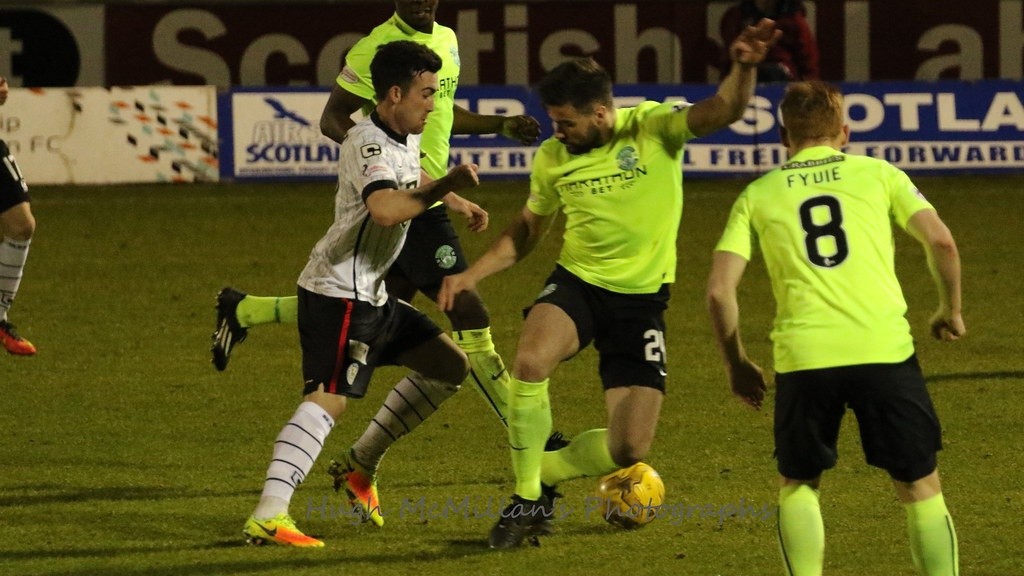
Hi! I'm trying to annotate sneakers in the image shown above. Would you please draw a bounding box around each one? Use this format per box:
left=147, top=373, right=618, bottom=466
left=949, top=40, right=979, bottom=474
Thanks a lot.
left=243, top=512, right=325, bottom=551
left=210, top=287, right=248, bottom=373
left=329, top=451, right=383, bottom=526
left=0, top=321, right=38, bottom=356
left=490, top=492, right=560, bottom=548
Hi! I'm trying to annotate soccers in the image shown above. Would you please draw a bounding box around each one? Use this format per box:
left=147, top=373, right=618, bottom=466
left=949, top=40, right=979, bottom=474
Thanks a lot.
left=599, top=462, right=666, bottom=529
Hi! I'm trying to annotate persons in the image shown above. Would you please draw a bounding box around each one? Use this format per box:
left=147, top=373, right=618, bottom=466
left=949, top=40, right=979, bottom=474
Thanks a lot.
left=704, top=76, right=969, bottom=576
left=0, top=77, right=41, bottom=359
left=209, top=0, right=578, bottom=451
left=233, top=39, right=490, bottom=549
left=719, top=0, right=820, bottom=80
left=436, top=21, right=780, bottom=551
